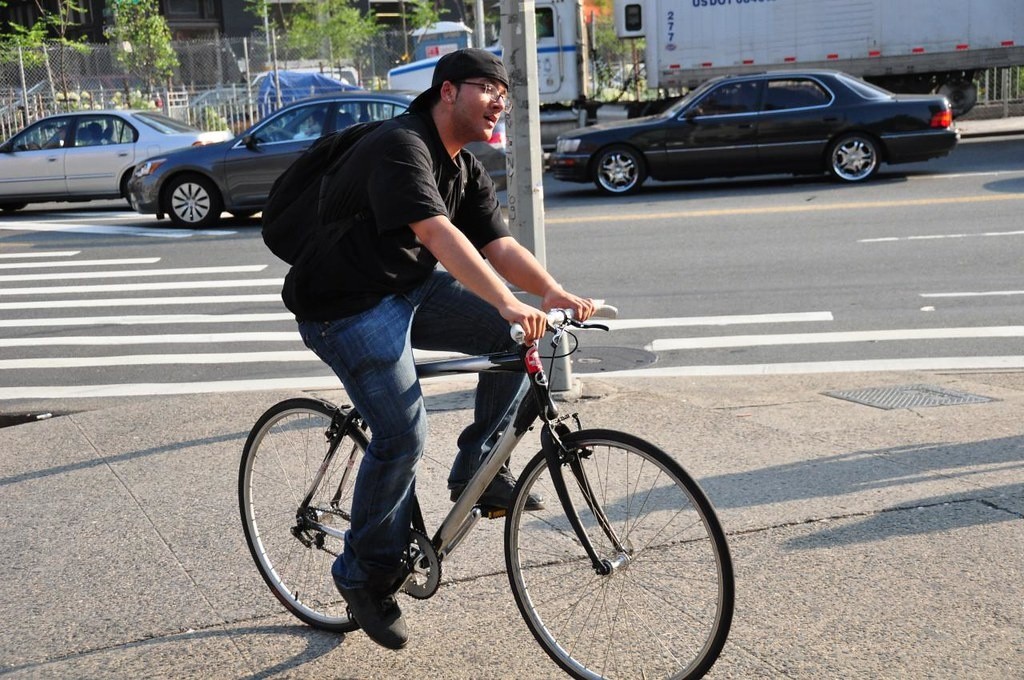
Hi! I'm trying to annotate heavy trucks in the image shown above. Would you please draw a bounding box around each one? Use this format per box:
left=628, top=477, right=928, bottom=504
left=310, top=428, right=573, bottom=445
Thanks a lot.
left=387, top=0, right=1024, bottom=149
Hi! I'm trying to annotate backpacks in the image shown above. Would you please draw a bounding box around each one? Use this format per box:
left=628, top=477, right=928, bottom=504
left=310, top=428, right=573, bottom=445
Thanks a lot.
left=262, top=117, right=438, bottom=265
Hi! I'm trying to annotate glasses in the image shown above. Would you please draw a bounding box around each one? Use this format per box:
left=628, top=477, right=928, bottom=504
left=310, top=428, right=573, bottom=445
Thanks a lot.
left=455, top=80, right=509, bottom=107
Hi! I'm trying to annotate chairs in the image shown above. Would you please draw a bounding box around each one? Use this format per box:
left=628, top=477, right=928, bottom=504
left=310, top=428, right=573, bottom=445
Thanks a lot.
left=77, top=128, right=92, bottom=145
left=337, top=112, right=356, bottom=131
left=739, top=85, right=761, bottom=111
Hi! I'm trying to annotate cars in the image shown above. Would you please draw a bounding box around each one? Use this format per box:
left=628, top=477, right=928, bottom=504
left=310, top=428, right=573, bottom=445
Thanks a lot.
left=547, top=69, right=959, bottom=198
left=126, top=92, right=506, bottom=229
left=0, top=109, right=235, bottom=210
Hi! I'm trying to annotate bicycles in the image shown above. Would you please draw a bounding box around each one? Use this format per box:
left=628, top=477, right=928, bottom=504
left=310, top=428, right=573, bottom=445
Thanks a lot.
left=238, top=297, right=735, bottom=680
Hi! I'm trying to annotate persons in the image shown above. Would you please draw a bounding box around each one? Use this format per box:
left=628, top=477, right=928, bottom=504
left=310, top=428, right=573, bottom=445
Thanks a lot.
left=282, top=49, right=597, bottom=649
left=26, top=123, right=103, bottom=151
left=268, top=111, right=324, bottom=141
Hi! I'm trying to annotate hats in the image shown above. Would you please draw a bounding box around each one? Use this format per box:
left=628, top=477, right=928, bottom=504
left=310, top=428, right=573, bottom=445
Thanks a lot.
left=409, top=48, right=509, bottom=115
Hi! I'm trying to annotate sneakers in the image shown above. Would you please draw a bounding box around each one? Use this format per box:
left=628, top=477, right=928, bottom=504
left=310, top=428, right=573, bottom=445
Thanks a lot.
left=450, top=468, right=544, bottom=510
left=340, top=581, right=409, bottom=648
